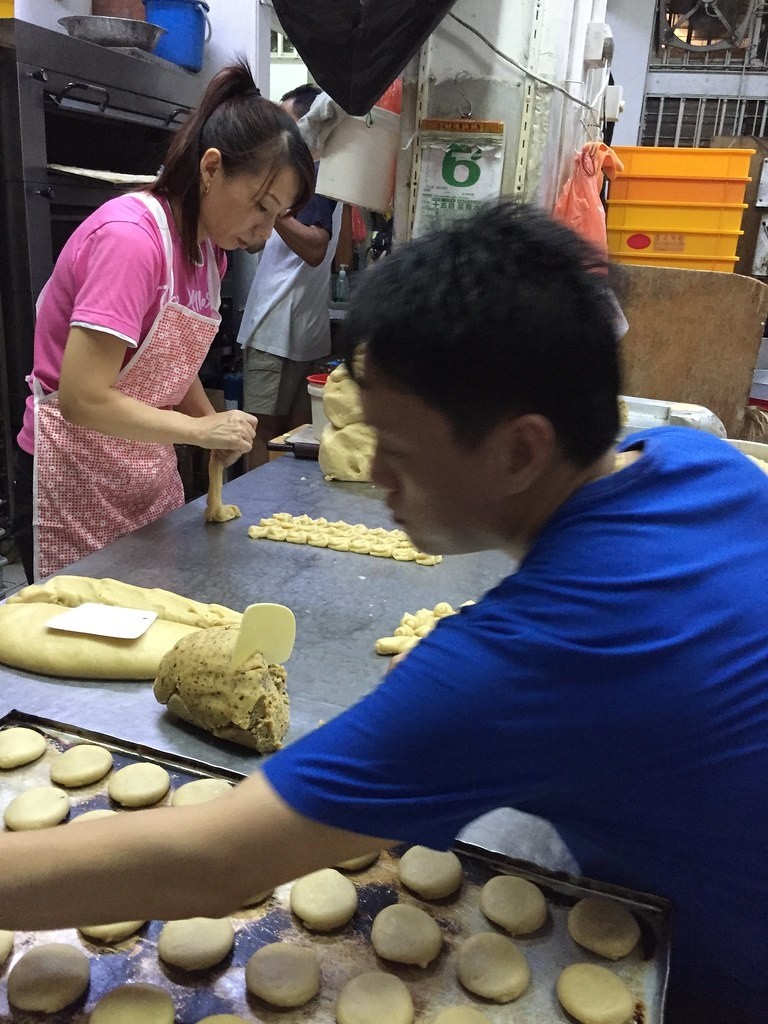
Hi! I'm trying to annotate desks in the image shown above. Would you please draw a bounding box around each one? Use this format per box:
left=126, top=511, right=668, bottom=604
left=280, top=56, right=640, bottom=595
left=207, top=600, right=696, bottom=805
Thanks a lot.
left=0, top=454, right=576, bottom=889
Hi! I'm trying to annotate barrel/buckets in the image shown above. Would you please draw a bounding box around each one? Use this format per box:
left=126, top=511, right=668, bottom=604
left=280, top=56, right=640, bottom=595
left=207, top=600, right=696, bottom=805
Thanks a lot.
left=141, top=1, right=211, bottom=72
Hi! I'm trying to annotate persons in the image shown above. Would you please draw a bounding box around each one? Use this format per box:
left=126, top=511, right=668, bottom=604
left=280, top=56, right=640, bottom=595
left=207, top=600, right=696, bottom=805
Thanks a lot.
left=11, top=57, right=316, bottom=587
left=1, top=197, right=766, bottom=1023
left=236, top=83, right=345, bottom=453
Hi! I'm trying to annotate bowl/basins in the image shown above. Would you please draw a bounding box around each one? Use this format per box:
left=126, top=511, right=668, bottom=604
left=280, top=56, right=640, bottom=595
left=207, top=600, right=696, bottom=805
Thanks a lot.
left=58, top=16, right=169, bottom=53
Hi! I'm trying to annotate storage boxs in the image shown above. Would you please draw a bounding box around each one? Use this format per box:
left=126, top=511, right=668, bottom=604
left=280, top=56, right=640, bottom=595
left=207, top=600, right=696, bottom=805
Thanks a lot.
left=606, top=250, right=741, bottom=276
left=606, top=172, right=753, bottom=205
left=604, top=226, right=745, bottom=257
left=609, top=144, right=756, bottom=179
left=602, top=198, right=748, bottom=232
